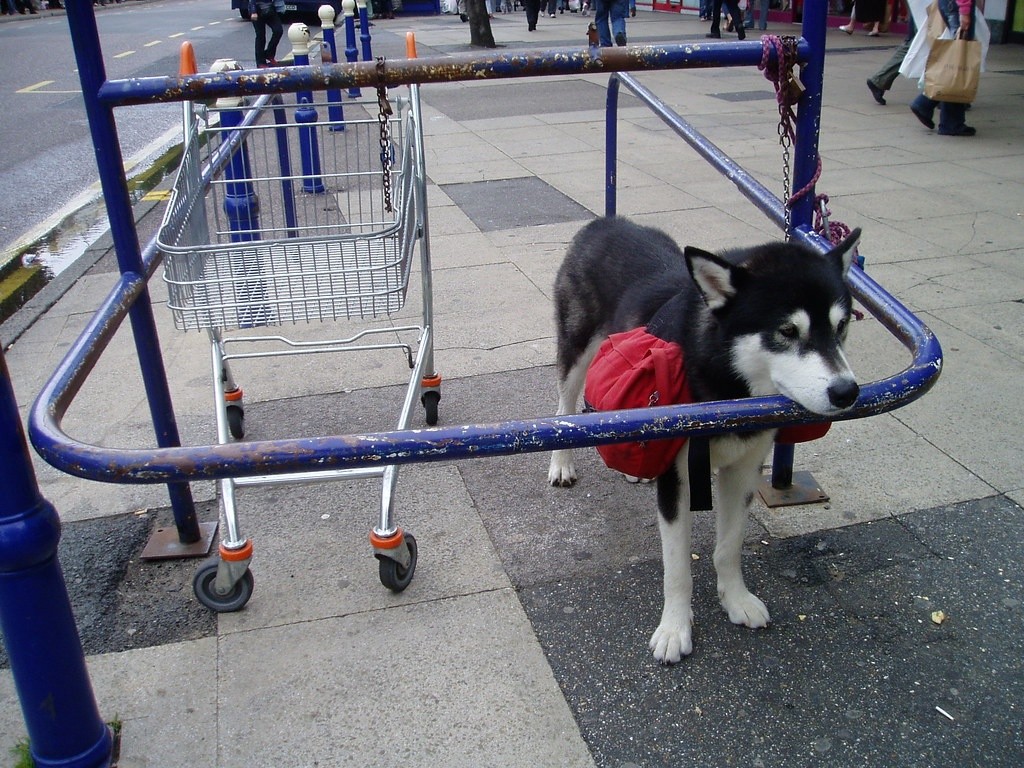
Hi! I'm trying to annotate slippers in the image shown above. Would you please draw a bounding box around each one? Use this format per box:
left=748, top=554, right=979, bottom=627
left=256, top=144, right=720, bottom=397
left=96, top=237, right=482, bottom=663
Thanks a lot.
left=839, top=25, right=852, bottom=35
left=868, top=31, right=879, bottom=36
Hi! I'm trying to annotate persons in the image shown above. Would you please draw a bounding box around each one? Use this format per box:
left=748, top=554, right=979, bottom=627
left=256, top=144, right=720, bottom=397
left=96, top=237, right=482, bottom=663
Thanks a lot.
left=458, top=0, right=636, bottom=47
left=248, top=0, right=286, bottom=68
left=0, top=0, right=124, bottom=14
left=699, top=0, right=769, bottom=40
left=838, top=0, right=979, bottom=136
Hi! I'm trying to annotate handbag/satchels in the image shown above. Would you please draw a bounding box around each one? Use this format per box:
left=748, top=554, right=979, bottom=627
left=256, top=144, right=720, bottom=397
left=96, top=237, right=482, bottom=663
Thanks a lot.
left=585, top=327, right=695, bottom=478
left=772, top=422, right=831, bottom=444
left=923, top=27, right=982, bottom=103
left=738, top=0, right=750, bottom=12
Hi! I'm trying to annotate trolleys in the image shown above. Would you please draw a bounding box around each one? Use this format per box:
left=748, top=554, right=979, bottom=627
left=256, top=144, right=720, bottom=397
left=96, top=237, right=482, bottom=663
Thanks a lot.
left=153, top=30, right=443, bottom=613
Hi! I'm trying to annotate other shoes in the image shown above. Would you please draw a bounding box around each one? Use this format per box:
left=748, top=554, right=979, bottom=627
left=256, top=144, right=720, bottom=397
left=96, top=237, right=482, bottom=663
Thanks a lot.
left=727, top=18, right=735, bottom=32
left=550, top=13, right=555, bottom=18
left=744, top=27, right=753, bottom=30
left=909, top=105, right=935, bottom=130
left=867, top=78, right=886, bottom=106
left=632, top=10, right=636, bottom=17
left=269, top=57, right=280, bottom=67
left=706, top=33, right=721, bottom=38
left=616, top=34, right=626, bottom=46
left=257, top=63, right=271, bottom=68
left=738, top=26, right=745, bottom=40
left=701, top=16, right=707, bottom=21
left=938, top=127, right=976, bottom=136
left=540, top=10, right=545, bottom=17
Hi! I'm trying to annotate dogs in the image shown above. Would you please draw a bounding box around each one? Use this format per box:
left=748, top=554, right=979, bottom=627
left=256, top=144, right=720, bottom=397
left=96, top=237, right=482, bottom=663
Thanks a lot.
left=547, top=216, right=862, bottom=666
